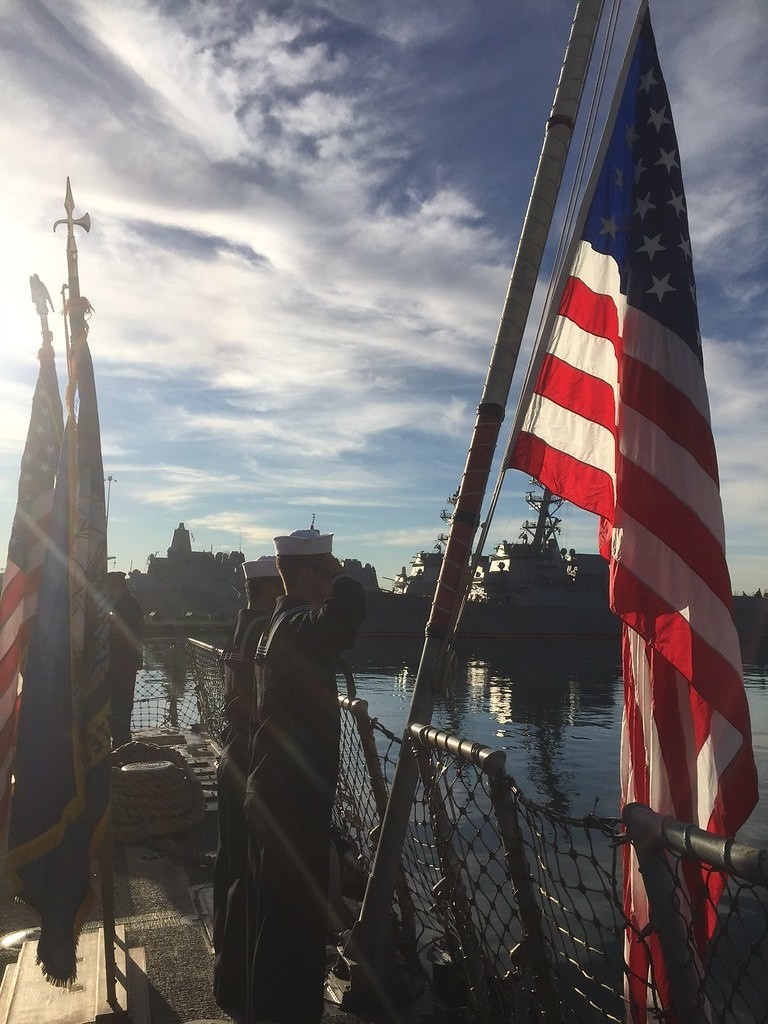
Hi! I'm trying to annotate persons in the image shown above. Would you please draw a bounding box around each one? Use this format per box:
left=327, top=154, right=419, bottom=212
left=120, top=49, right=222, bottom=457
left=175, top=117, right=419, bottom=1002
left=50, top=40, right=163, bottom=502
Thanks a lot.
left=213, top=530, right=366, bottom=1024
left=106, top=572, right=145, bottom=750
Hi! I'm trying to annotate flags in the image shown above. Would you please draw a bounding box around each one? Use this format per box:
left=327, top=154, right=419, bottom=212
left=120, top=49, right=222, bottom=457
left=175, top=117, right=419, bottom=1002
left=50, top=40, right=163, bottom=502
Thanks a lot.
left=506, top=0, right=760, bottom=1024
left=0, top=296, right=112, bottom=990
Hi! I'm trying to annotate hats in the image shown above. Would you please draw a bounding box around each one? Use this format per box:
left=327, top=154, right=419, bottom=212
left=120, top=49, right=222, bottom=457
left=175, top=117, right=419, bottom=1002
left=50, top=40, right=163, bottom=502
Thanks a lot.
left=274, top=530, right=334, bottom=556
left=241, top=556, right=281, bottom=580
left=107, top=572, right=126, bottom=580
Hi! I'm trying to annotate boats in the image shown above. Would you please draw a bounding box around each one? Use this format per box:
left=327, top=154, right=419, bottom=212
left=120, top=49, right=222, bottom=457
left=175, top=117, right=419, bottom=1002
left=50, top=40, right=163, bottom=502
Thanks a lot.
left=125, top=476, right=623, bottom=667
